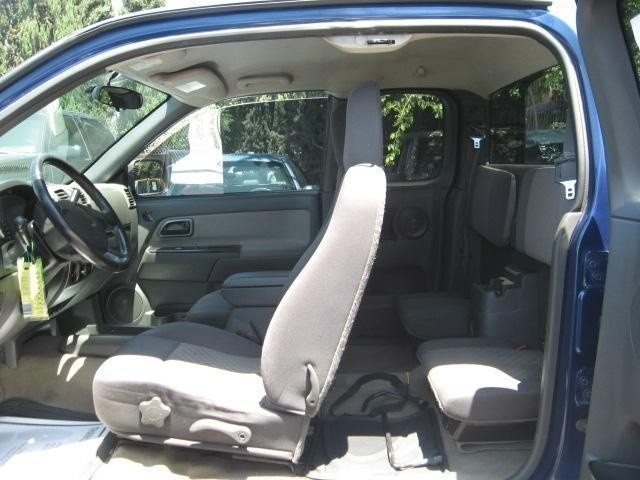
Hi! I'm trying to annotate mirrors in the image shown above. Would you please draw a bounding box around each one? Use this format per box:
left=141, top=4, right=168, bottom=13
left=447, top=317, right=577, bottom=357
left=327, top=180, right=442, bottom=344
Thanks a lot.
left=97, top=85, right=144, bottom=109
